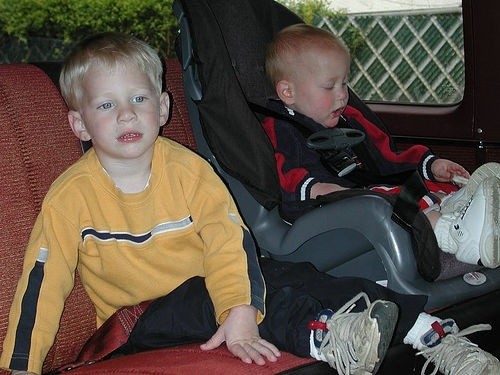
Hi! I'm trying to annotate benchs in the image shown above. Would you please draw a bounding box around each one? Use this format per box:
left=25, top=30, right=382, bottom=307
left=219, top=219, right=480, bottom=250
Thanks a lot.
left=0, top=57, right=500, bottom=375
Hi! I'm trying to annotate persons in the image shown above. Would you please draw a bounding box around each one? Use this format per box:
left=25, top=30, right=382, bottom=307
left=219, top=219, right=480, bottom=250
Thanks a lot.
left=0, top=30, right=500, bottom=375
left=261, top=24, right=499, bottom=268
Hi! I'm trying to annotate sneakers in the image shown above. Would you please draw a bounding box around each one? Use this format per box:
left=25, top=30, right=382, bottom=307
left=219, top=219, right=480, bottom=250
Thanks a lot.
left=308, top=292, right=399, bottom=375
left=440, top=162, right=500, bottom=222
left=449, top=176, right=500, bottom=269
left=416, top=318, right=500, bottom=375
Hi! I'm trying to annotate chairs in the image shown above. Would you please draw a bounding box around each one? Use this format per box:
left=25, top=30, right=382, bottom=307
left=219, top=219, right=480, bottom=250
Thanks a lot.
left=172, top=1, right=500, bottom=346
